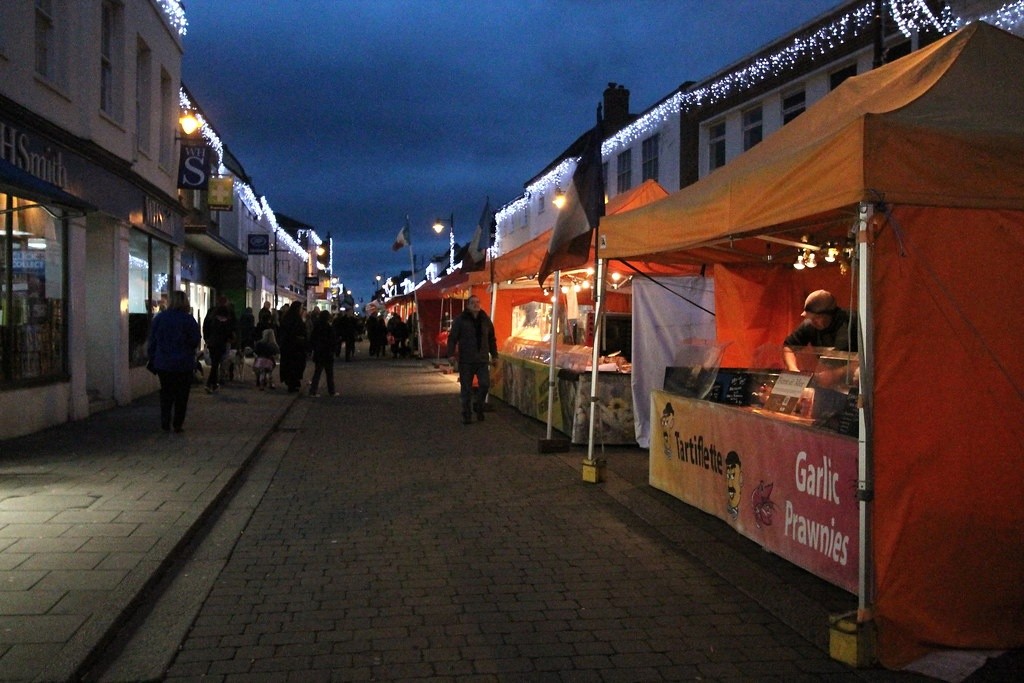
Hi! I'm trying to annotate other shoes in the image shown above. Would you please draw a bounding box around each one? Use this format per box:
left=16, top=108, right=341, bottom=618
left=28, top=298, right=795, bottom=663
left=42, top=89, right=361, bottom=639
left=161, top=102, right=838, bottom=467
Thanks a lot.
left=461, top=411, right=473, bottom=424
left=472, top=401, right=485, bottom=421
left=162, top=422, right=185, bottom=434
left=309, top=393, right=320, bottom=398
left=204, top=382, right=221, bottom=394
left=330, top=392, right=340, bottom=397
left=259, top=383, right=276, bottom=391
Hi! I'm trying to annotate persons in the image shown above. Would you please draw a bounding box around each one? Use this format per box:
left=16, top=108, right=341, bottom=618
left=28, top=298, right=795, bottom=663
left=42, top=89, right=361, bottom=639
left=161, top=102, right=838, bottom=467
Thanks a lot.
left=147, top=291, right=199, bottom=432
left=280, top=301, right=305, bottom=393
left=254, top=329, right=280, bottom=390
left=204, top=305, right=230, bottom=393
left=447, top=295, right=498, bottom=423
left=214, top=297, right=416, bottom=361
left=309, top=311, right=339, bottom=398
left=783, top=290, right=860, bottom=384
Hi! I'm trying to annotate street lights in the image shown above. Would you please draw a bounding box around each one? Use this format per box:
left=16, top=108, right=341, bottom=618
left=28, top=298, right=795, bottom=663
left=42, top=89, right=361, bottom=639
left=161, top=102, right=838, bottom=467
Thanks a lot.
left=340, top=272, right=382, bottom=316
left=431, top=212, right=456, bottom=274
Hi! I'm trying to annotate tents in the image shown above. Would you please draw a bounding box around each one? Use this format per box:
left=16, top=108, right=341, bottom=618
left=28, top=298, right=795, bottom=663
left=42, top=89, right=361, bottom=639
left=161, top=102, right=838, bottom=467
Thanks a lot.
left=491, top=177, right=670, bottom=444
left=583, top=20, right=1022, bottom=664
left=385, top=269, right=490, bottom=360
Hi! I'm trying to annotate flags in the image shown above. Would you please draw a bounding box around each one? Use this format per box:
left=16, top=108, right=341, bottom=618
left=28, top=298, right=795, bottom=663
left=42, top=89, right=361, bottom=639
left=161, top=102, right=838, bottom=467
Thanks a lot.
left=462, top=201, right=489, bottom=270
left=392, top=221, right=410, bottom=252
left=537, top=124, right=603, bottom=287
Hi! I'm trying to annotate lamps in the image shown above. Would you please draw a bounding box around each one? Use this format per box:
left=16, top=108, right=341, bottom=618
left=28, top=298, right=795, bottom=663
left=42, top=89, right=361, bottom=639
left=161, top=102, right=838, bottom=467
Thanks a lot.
left=805, top=249, right=818, bottom=268
left=825, top=240, right=838, bottom=263
left=180, top=109, right=199, bottom=134
left=843, top=238, right=855, bottom=259
left=792, top=251, right=806, bottom=271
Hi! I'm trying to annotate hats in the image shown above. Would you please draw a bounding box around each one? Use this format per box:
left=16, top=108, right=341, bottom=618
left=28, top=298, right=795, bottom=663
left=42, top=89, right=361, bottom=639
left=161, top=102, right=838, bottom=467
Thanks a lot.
left=800, top=289, right=838, bottom=319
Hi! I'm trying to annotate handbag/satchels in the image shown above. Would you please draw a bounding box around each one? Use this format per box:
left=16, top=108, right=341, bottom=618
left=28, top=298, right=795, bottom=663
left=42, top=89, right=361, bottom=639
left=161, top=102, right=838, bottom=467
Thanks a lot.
left=146, top=340, right=157, bottom=375
